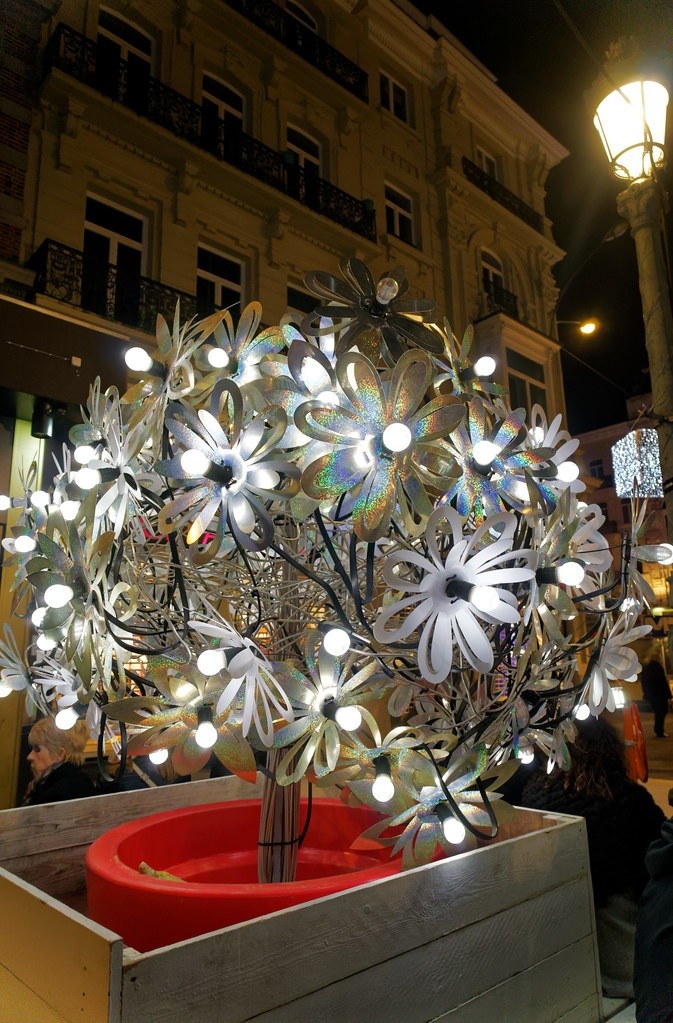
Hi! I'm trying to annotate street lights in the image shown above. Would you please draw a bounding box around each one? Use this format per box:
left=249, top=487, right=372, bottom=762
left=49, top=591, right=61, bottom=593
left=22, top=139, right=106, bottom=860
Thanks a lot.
left=586, top=30, right=673, bottom=501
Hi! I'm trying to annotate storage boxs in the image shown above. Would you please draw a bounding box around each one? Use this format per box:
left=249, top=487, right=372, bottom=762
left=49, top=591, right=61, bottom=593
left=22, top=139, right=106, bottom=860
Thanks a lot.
left=1, top=769, right=603, bottom=1023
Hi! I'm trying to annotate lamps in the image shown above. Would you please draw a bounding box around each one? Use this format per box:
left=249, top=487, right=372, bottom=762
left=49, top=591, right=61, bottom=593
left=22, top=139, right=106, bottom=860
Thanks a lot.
left=0, top=256, right=673, bottom=873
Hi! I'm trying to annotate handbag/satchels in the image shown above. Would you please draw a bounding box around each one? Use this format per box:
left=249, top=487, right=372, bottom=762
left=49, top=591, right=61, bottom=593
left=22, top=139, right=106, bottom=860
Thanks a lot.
left=637, top=699, right=653, bottom=712
left=623, top=703, right=648, bottom=783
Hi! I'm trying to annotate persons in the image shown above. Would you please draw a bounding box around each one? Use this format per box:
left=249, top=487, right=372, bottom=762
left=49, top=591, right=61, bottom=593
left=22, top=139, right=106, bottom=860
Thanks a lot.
left=498, top=713, right=673, bottom=1023
left=24, top=714, right=96, bottom=807
left=639, top=642, right=673, bottom=737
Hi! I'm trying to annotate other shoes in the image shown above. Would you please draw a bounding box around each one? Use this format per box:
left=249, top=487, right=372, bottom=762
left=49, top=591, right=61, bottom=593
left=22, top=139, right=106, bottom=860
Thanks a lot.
left=657, top=733, right=669, bottom=738
